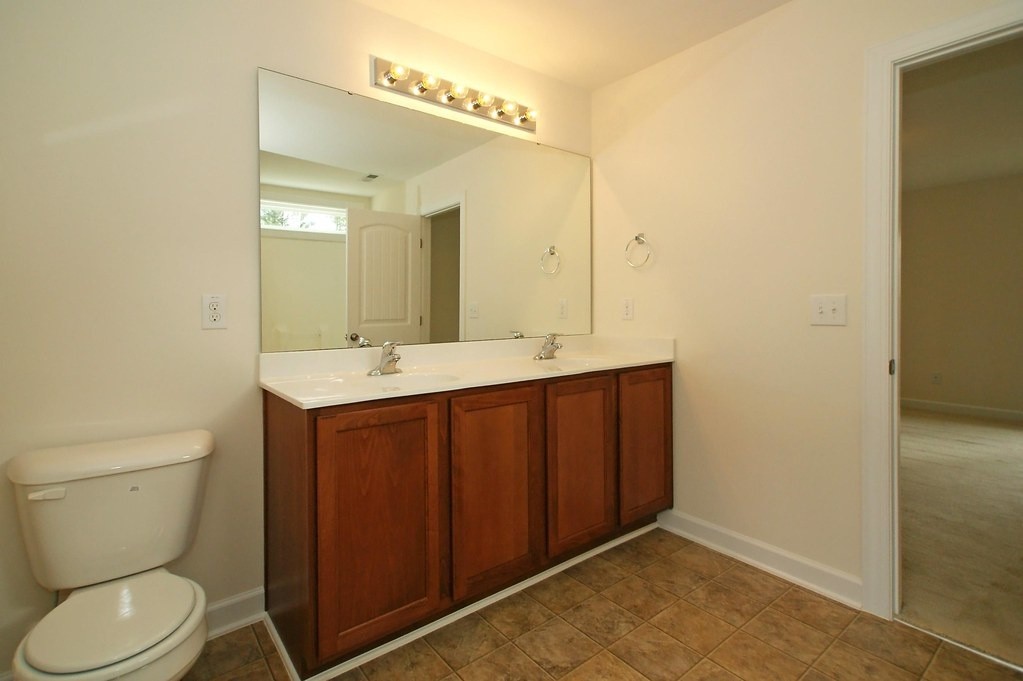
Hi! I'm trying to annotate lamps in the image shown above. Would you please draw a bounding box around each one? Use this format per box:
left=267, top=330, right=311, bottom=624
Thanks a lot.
left=373, top=58, right=537, bottom=131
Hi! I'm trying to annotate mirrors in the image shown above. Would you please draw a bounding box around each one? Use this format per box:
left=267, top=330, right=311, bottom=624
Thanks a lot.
left=255, top=65, right=593, bottom=353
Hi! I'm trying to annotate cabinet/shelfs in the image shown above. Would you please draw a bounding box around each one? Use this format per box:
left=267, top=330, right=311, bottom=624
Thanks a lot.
left=545, top=360, right=673, bottom=572
left=262, top=379, right=546, bottom=681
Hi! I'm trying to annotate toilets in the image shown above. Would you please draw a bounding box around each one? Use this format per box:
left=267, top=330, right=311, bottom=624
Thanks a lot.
left=6, top=430, right=214, bottom=681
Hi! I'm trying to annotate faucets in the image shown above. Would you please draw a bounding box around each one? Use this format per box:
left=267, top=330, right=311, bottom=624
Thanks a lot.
left=358, top=337, right=371, bottom=347
left=533, top=333, right=563, bottom=360
left=367, top=342, right=401, bottom=376
left=510, top=331, right=524, bottom=338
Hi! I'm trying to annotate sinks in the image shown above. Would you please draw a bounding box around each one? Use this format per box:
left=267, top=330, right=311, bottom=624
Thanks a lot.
left=501, top=356, right=618, bottom=376
left=329, top=374, right=459, bottom=392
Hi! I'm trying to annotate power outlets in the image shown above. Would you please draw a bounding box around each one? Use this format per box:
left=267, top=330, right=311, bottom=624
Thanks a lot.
left=201, top=293, right=228, bottom=330
left=931, top=371, right=943, bottom=385
left=557, top=299, right=568, bottom=319
left=620, top=296, right=633, bottom=319
